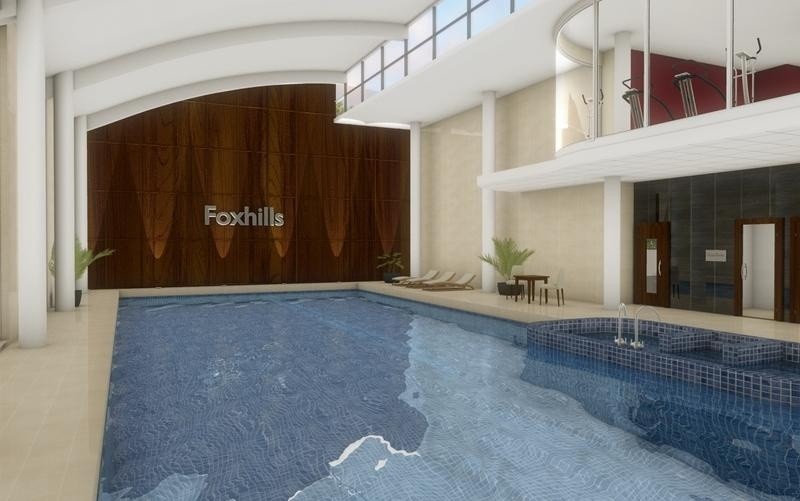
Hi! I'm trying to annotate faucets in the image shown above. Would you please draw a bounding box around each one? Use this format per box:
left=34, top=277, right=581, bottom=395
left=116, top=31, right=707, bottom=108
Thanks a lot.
left=614, top=303, right=628, bottom=346
left=630, top=305, right=662, bottom=349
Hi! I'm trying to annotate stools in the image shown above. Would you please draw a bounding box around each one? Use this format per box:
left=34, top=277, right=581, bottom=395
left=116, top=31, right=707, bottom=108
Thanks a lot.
left=497, top=282, right=525, bottom=299
left=537, top=283, right=565, bottom=307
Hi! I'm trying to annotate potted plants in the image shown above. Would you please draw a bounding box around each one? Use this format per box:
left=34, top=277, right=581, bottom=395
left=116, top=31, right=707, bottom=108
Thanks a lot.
left=377, top=252, right=403, bottom=283
left=49, top=232, right=113, bottom=306
left=479, top=237, right=536, bottom=295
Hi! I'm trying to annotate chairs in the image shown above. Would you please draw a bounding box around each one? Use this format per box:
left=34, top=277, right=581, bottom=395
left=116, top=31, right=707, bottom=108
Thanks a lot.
left=392, top=270, right=476, bottom=290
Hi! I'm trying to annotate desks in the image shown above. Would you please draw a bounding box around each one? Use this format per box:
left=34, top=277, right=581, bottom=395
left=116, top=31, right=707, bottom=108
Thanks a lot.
left=513, top=275, right=550, bottom=304
